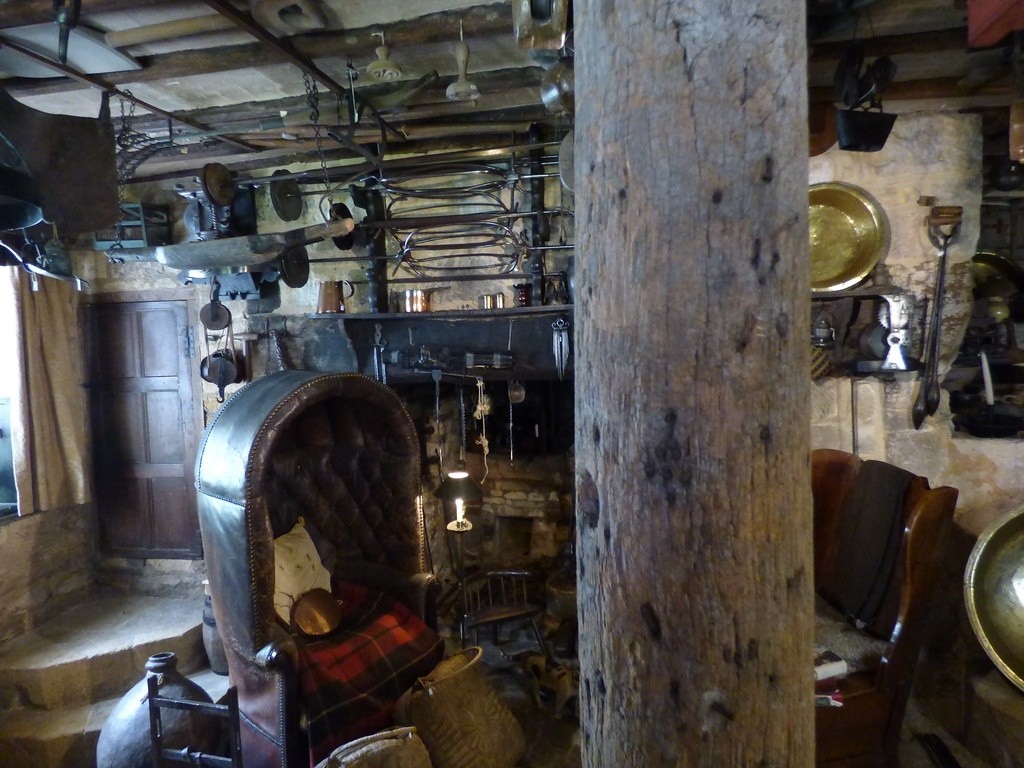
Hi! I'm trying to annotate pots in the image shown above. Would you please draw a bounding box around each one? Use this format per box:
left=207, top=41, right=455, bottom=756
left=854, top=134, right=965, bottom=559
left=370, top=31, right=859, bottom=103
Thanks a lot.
left=834, top=105, right=897, bottom=152
left=397, top=286, right=455, bottom=312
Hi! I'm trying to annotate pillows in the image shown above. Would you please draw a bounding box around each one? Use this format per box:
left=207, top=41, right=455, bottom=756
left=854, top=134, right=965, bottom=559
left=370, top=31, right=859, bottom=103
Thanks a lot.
left=273, top=516, right=332, bottom=625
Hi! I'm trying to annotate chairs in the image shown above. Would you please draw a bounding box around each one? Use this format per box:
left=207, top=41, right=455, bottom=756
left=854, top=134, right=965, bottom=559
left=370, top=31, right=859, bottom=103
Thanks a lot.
left=147, top=675, right=243, bottom=768
left=457, top=569, right=548, bottom=655
left=193, top=370, right=447, bottom=768
left=809, top=447, right=958, bottom=768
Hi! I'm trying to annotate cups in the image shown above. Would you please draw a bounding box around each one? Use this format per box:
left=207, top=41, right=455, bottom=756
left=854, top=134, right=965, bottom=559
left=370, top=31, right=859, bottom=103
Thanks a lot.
left=482, top=293, right=503, bottom=309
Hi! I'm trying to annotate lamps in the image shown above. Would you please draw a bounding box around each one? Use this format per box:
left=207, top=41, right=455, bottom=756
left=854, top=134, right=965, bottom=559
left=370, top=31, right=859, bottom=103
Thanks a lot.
left=446, top=445, right=469, bottom=480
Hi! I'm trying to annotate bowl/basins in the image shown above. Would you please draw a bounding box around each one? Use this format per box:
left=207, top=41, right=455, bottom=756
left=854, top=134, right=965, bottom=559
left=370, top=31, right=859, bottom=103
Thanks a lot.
left=965, top=503, right=1023, bottom=692
left=974, top=251, right=1022, bottom=315
left=807, top=182, right=886, bottom=291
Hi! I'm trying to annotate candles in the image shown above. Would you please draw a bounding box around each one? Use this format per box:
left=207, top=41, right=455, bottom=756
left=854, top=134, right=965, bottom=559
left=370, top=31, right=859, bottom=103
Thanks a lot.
left=455, top=498, right=464, bottom=522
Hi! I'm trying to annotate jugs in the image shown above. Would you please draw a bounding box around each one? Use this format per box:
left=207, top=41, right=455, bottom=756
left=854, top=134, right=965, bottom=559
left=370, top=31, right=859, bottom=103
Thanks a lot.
left=317, top=279, right=355, bottom=313
